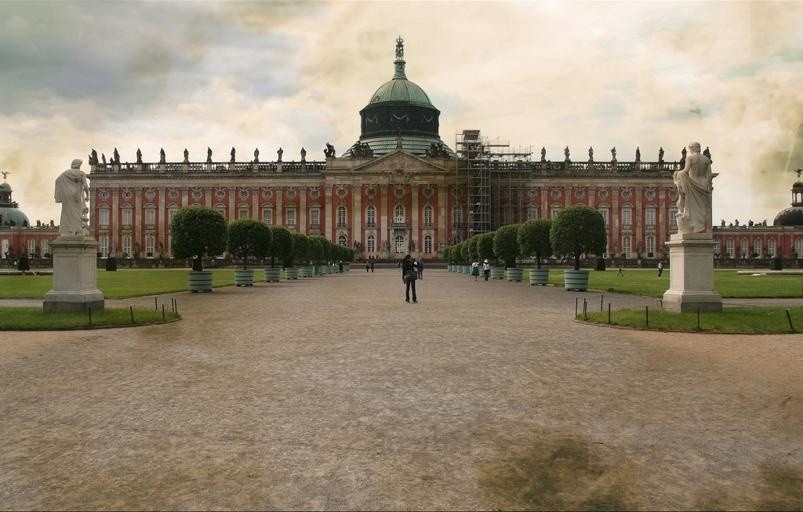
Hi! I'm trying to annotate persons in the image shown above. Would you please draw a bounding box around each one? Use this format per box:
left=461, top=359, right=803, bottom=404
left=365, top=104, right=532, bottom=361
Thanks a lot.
left=672, top=142, right=713, bottom=237
left=365, top=260, right=369, bottom=272
left=482, top=259, right=489, bottom=281
left=470, top=259, right=479, bottom=282
left=370, top=260, right=374, bottom=272
left=401, top=254, right=417, bottom=304
left=339, top=260, right=342, bottom=272
left=417, top=257, right=422, bottom=280
left=657, top=259, right=663, bottom=277
left=52, top=158, right=88, bottom=238
left=615, top=262, right=624, bottom=278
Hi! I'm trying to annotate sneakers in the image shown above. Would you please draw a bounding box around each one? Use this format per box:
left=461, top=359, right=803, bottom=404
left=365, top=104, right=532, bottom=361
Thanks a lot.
left=405, top=299, right=418, bottom=303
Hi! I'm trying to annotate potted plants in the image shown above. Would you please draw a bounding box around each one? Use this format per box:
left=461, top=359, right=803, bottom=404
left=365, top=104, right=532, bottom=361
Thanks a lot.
left=443, top=204, right=607, bottom=292
left=168, top=204, right=354, bottom=293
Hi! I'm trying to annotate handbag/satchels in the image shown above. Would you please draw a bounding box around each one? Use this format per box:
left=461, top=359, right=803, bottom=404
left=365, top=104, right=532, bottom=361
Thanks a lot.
left=403, top=269, right=418, bottom=283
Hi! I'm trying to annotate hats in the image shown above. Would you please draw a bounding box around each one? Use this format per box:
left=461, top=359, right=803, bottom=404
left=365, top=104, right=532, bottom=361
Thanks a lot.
left=483, top=259, right=489, bottom=264
